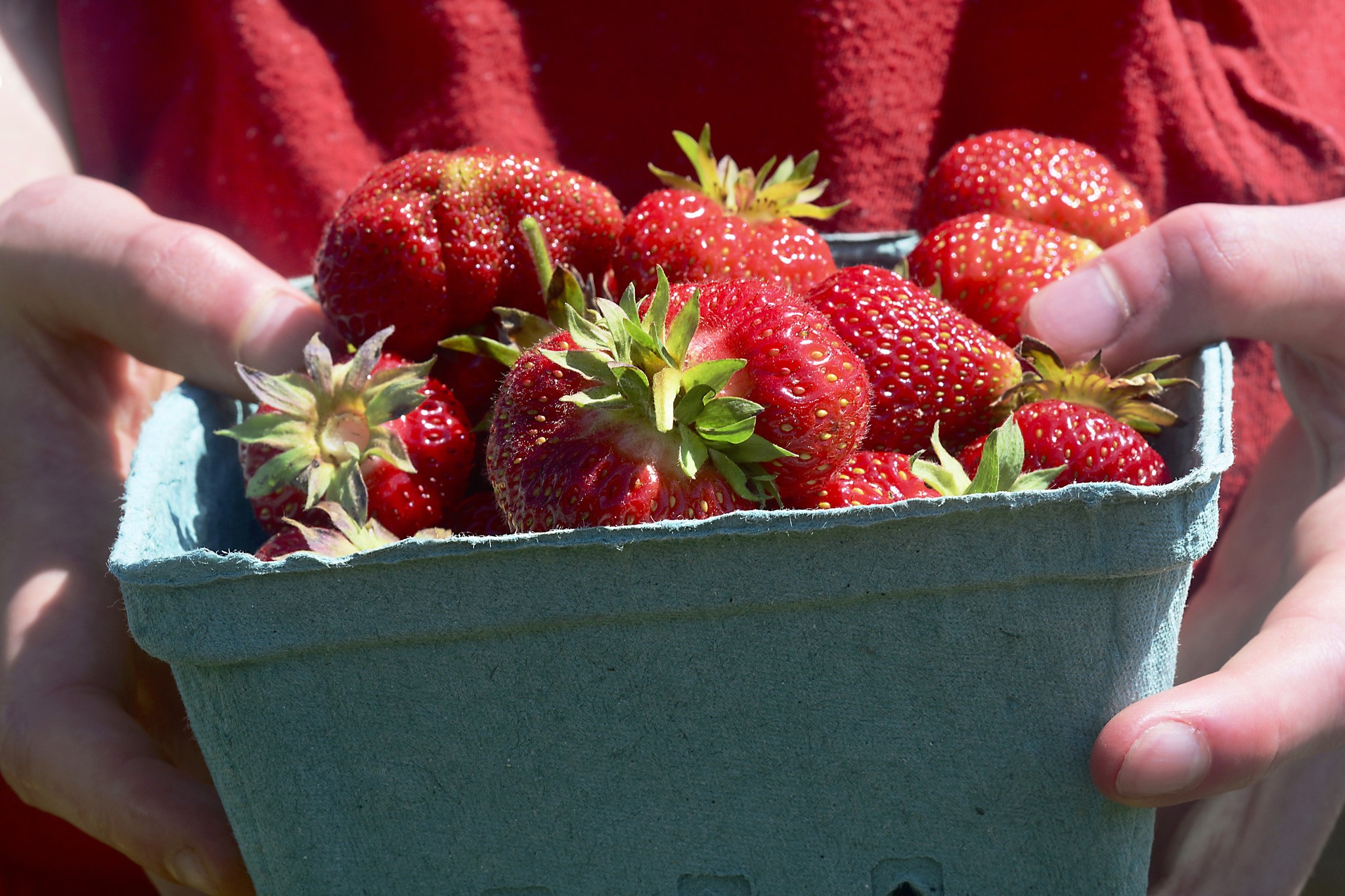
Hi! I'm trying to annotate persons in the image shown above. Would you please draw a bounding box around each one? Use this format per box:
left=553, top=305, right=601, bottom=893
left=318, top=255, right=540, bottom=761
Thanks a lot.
left=0, top=0, right=1345, bottom=896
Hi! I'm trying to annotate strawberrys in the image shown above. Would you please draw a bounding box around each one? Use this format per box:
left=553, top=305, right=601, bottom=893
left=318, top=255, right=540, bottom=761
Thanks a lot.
left=212, top=126, right=1201, bottom=551
